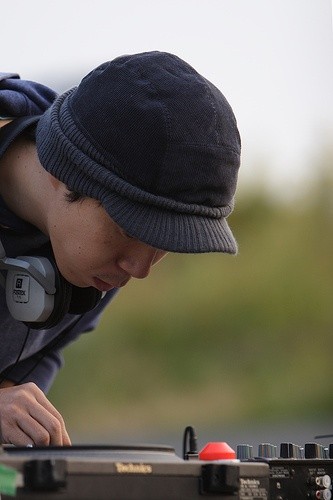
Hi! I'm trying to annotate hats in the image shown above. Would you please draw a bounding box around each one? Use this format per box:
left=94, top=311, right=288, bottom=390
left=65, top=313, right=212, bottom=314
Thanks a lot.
left=35, top=50, right=242, bottom=255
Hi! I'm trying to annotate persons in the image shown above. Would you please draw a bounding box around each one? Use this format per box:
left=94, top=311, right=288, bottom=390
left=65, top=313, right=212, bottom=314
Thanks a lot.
left=0, top=51, right=241, bottom=448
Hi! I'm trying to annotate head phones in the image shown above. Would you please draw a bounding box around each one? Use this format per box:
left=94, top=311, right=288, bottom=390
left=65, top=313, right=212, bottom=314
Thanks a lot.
left=0, top=236, right=102, bottom=330
